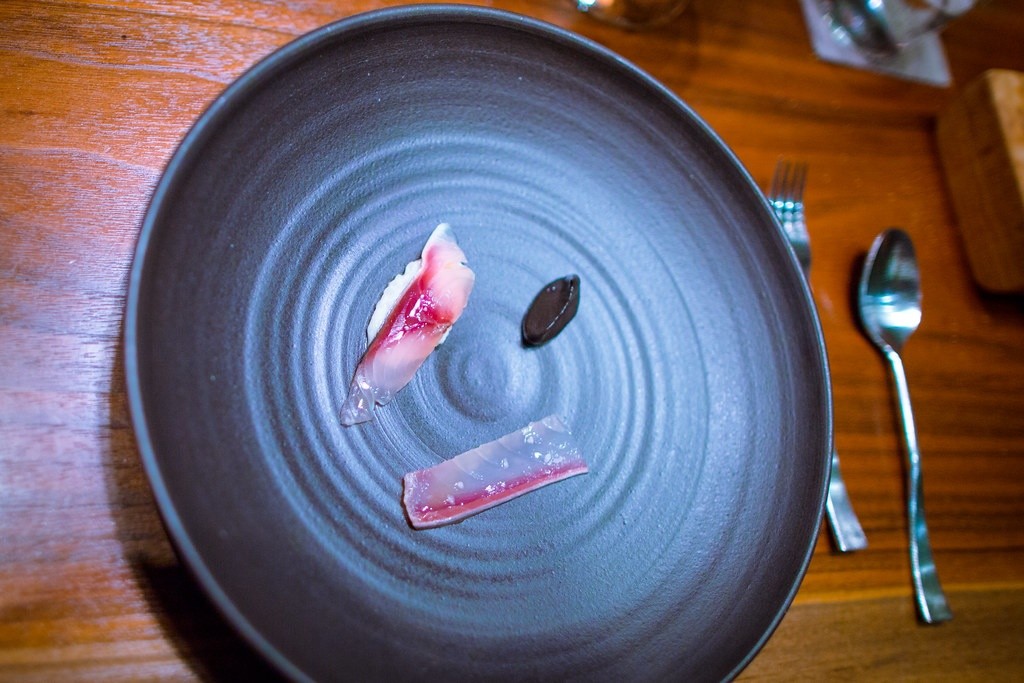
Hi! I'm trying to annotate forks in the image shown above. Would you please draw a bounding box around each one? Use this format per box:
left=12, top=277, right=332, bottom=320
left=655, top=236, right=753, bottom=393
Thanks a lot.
left=767, top=157, right=871, bottom=552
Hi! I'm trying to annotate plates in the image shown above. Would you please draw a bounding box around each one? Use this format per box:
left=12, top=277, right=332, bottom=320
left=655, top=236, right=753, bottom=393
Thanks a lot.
left=122, top=3, right=833, bottom=683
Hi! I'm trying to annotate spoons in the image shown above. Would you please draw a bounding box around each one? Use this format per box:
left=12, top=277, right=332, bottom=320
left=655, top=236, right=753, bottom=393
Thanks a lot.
left=857, top=225, right=955, bottom=624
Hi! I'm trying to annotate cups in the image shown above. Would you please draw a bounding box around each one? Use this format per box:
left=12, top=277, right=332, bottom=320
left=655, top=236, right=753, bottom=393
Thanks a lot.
left=811, top=0, right=979, bottom=58
